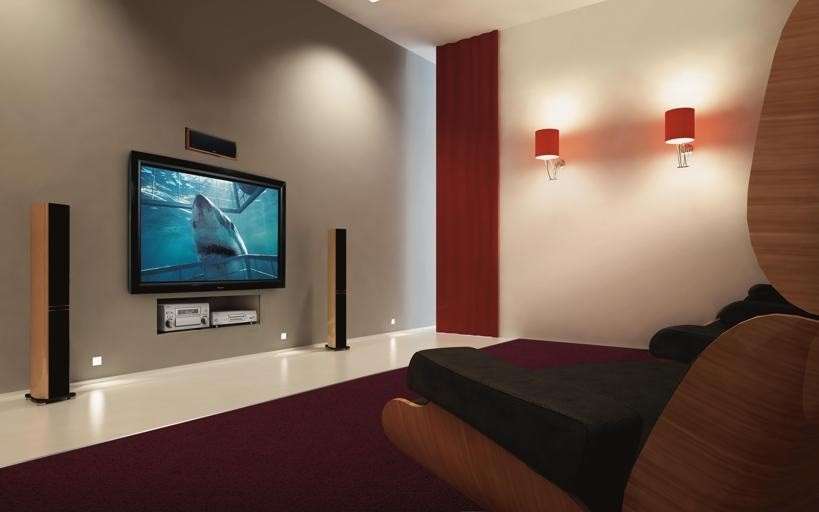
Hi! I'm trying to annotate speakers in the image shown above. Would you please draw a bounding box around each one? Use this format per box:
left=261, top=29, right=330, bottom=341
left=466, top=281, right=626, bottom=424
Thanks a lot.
left=24, top=199, right=78, bottom=402
left=183, top=125, right=239, bottom=158
left=323, top=226, right=354, bottom=352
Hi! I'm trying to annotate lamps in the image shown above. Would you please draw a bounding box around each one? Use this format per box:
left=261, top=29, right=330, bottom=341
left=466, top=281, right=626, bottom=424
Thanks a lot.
left=535, top=128, right=565, bottom=181
left=663, top=107, right=696, bottom=169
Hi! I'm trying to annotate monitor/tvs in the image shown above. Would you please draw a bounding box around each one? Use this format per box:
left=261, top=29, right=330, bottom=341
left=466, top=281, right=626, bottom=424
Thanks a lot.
left=126, top=148, right=288, bottom=291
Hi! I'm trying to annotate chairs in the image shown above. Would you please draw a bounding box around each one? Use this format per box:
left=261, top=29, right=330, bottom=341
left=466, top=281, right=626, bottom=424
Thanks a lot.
left=378, top=0, right=819, bottom=511
